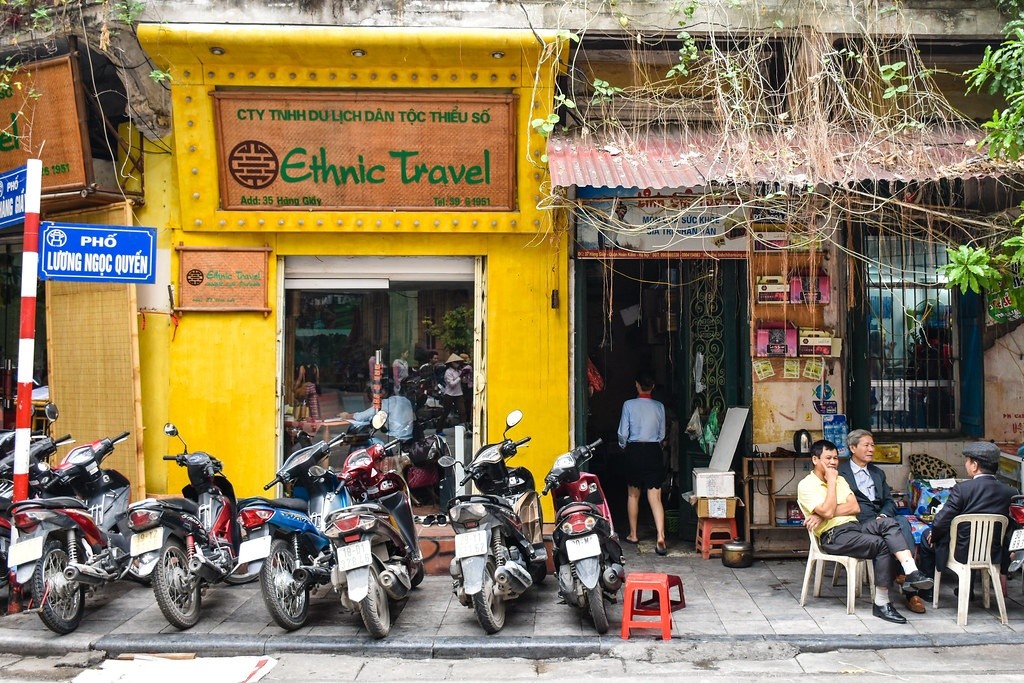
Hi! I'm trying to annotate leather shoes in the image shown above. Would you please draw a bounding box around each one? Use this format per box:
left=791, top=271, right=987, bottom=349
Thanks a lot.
left=873, top=603, right=906, bottom=623
left=902, top=571, right=934, bottom=592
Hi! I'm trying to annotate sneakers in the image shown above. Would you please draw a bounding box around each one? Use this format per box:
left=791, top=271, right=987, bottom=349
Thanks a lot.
left=896, top=575, right=906, bottom=584
left=905, top=595, right=926, bottom=613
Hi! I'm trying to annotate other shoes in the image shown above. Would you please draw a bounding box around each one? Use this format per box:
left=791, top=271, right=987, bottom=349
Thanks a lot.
left=954, top=588, right=975, bottom=601
left=435, top=431, right=446, bottom=436
left=919, top=590, right=933, bottom=602
left=465, top=430, right=473, bottom=435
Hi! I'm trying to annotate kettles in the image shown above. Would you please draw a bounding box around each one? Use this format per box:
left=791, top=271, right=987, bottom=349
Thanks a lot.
left=794, top=428, right=812, bottom=457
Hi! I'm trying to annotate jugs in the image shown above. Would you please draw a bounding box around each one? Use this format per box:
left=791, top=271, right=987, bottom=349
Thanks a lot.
left=930, top=502, right=944, bottom=515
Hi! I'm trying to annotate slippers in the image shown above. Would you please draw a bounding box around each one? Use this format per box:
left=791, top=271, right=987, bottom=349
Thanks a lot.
left=655, top=543, right=668, bottom=555
left=624, top=535, right=639, bottom=544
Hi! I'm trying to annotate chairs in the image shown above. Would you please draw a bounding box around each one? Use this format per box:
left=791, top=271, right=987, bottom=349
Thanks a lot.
left=933, top=513, right=1008, bottom=626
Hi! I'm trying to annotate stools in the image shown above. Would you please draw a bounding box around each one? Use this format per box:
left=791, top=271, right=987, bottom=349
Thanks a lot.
left=695, top=518, right=738, bottom=559
left=636, top=574, right=686, bottom=613
left=797, top=501, right=874, bottom=615
left=621, top=571, right=673, bottom=639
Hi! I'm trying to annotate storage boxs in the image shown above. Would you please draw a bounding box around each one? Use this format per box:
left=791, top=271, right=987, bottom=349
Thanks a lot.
left=692, top=467, right=735, bottom=497
left=696, top=497, right=736, bottom=518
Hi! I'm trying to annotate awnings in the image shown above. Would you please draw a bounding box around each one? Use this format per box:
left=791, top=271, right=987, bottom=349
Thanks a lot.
left=547, top=123, right=1024, bottom=188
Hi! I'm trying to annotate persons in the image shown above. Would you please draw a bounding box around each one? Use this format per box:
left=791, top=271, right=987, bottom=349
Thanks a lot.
left=837, top=429, right=926, bottom=613
left=284, top=361, right=319, bottom=466
left=336, top=380, right=416, bottom=456
left=617, top=373, right=668, bottom=555
left=443, top=353, right=472, bottom=436
left=906, top=325, right=955, bottom=427
left=368, top=346, right=386, bottom=396
left=917, top=442, right=1020, bottom=601
left=419, top=351, right=447, bottom=436
left=460, top=354, right=473, bottom=423
left=870, top=332, right=897, bottom=423
left=797, top=439, right=934, bottom=623
left=392, top=346, right=409, bottom=395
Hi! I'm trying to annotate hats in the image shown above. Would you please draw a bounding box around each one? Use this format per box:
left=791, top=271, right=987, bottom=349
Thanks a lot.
left=962, top=441, right=1001, bottom=464
left=444, top=353, right=464, bottom=365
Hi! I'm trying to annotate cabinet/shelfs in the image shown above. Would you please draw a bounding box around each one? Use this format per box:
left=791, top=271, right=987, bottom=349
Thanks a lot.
left=751, top=250, right=831, bottom=361
left=742, top=457, right=814, bottom=558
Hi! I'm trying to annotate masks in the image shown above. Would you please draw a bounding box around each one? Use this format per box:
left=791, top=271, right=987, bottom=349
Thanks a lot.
left=453, top=363, right=460, bottom=368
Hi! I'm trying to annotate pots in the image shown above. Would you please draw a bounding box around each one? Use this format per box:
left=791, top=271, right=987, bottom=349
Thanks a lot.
left=722, top=537, right=753, bottom=568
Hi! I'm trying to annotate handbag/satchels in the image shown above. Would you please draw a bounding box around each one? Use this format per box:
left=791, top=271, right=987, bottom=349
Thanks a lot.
left=295, top=386, right=306, bottom=402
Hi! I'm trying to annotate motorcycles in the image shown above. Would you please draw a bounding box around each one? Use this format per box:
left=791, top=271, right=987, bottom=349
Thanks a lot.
left=127, top=423, right=262, bottom=631
left=1009, top=495, right=1024, bottom=593
left=309, top=411, right=425, bottom=639
left=542, top=438, right=626, bottom=634
left=236, top=430, right=346, bottom=632
left=5, top=431, right=131, bottom=636
left=0, top=402, right=72, bottom=588
left=438, top=409, right=548, bottom=633
